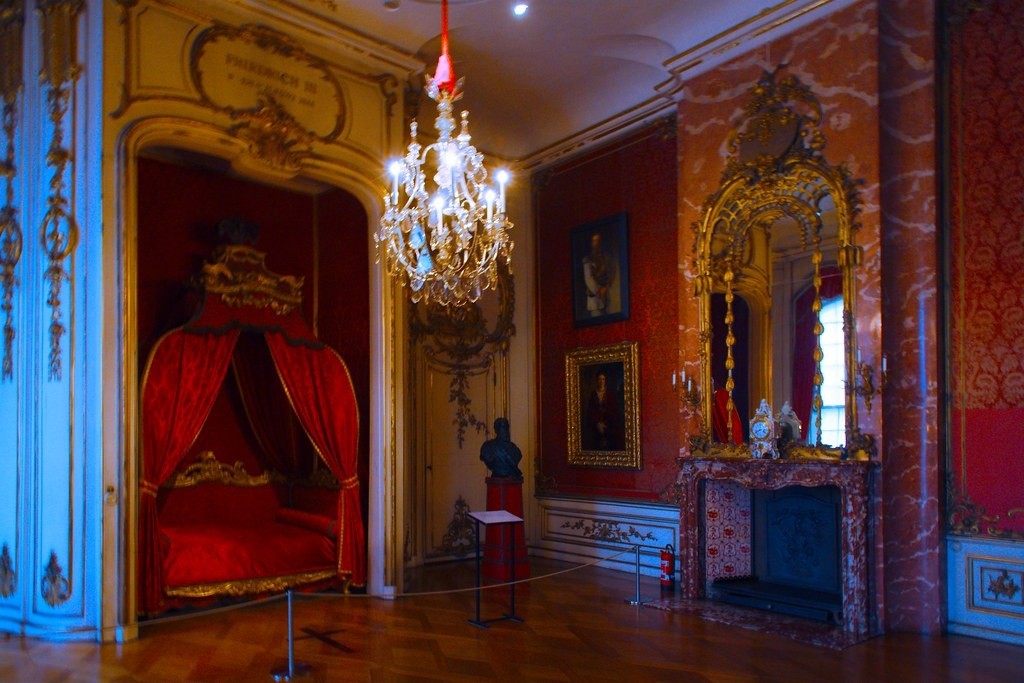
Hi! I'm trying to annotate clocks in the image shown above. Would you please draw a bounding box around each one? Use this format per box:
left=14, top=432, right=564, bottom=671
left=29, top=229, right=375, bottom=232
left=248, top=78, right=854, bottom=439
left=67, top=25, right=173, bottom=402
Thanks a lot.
left=776, top=401, right=801, bottom=445
left=749, top=398, right=780, bottom=460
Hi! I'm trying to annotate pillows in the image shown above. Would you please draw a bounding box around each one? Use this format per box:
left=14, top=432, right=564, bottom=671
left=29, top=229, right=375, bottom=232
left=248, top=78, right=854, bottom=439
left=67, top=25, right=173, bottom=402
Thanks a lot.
left=276, top=509, right=339, bottom=538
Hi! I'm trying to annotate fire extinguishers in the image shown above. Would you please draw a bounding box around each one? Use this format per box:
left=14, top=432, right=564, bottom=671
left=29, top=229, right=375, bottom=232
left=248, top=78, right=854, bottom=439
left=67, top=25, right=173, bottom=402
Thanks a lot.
left=660, top=544, right=675, bottom=592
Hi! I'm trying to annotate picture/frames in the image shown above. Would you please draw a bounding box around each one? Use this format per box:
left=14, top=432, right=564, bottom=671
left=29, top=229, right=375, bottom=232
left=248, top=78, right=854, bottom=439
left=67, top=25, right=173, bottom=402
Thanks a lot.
left=563, top=341, right=641, bottom=470
left=567, top=209, right=629, bottom=328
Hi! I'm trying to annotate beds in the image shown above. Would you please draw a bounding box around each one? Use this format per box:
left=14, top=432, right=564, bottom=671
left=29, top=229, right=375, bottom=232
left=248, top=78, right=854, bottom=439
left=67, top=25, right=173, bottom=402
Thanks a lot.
left=160, top=510, right=341, bottom=610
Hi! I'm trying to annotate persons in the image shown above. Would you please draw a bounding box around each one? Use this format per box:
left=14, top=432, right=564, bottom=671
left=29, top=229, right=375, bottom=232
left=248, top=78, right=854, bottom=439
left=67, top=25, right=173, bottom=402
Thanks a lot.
left=583, top=234, right=618, bottom=317
left=480, top=417, right=523, bottom=478
left=587, top=373, right=623, bottom=451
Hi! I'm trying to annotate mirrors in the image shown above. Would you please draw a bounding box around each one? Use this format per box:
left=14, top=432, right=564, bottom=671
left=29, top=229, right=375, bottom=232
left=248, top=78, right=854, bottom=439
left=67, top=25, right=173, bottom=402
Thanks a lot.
left=693, top=68, right=857, bottom=460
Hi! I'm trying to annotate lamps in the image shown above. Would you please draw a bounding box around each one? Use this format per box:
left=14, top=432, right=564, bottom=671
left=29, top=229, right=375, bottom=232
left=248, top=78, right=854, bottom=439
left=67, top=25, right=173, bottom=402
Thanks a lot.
left=374, top=0, right=514, bottom=305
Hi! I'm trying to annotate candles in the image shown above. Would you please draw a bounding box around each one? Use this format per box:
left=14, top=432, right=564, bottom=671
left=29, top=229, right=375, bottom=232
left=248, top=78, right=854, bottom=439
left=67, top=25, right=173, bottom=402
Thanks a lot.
left=858, top=350, right=862, bottom=363
left=688, top=380, right=692, bottom=391
left=672, top=373, right=676, bottom=384
left=681, top=370, right=686, bottom=382
left=883, top=357, right=887, bottom=371
